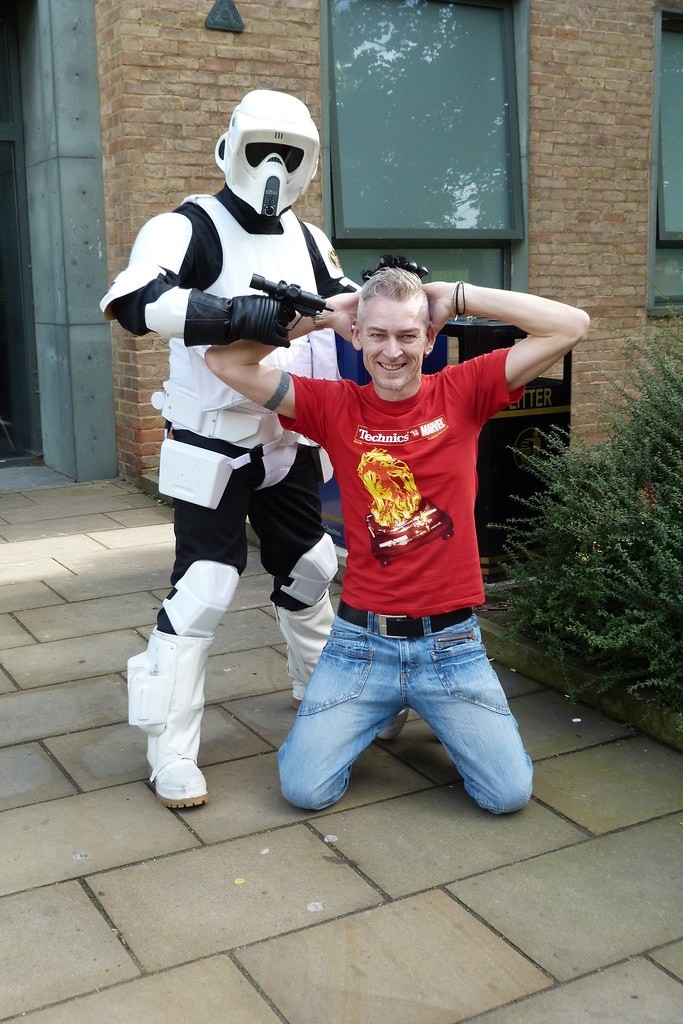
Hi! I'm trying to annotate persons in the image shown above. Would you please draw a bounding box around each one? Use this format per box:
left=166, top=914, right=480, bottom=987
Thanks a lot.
left=205, top=265, right=591, bottom=816
left=100, top=89, right=428, bottom=808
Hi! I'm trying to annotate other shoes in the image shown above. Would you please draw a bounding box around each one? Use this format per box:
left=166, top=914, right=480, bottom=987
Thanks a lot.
left=379, top=709, right=410, bottom=739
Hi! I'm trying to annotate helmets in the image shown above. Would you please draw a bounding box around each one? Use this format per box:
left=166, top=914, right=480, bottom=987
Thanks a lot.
left=215, top=89, right=320, bottom=217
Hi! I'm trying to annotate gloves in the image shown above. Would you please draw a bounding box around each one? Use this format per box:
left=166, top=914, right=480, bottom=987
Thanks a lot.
left=184, top=287, right=296, bottom=348
left=361, top=251, right=427, bottom=283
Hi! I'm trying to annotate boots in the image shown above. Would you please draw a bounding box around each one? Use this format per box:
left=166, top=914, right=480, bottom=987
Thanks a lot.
left=126, top=625, right=216, bottom=807
left=270, top=597, right=337, bottom=708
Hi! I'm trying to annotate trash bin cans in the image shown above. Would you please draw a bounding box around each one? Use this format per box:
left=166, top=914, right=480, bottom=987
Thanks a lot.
left=438, top=314, right=573, bottom=584
left=319, top=329, right=450, bottom=550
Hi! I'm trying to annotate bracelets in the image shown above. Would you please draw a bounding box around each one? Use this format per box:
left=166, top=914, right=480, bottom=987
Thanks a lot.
left=315, top=311, right=324, bottom=328
left=452, top=281, right=466, bottom=322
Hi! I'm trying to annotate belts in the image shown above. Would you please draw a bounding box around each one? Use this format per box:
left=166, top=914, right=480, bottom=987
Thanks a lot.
left=336, top=599, right=473, bottom=639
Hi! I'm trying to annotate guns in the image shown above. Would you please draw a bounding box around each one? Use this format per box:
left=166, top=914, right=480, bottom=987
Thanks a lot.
left=249, top=272, right=335, bottom=330
